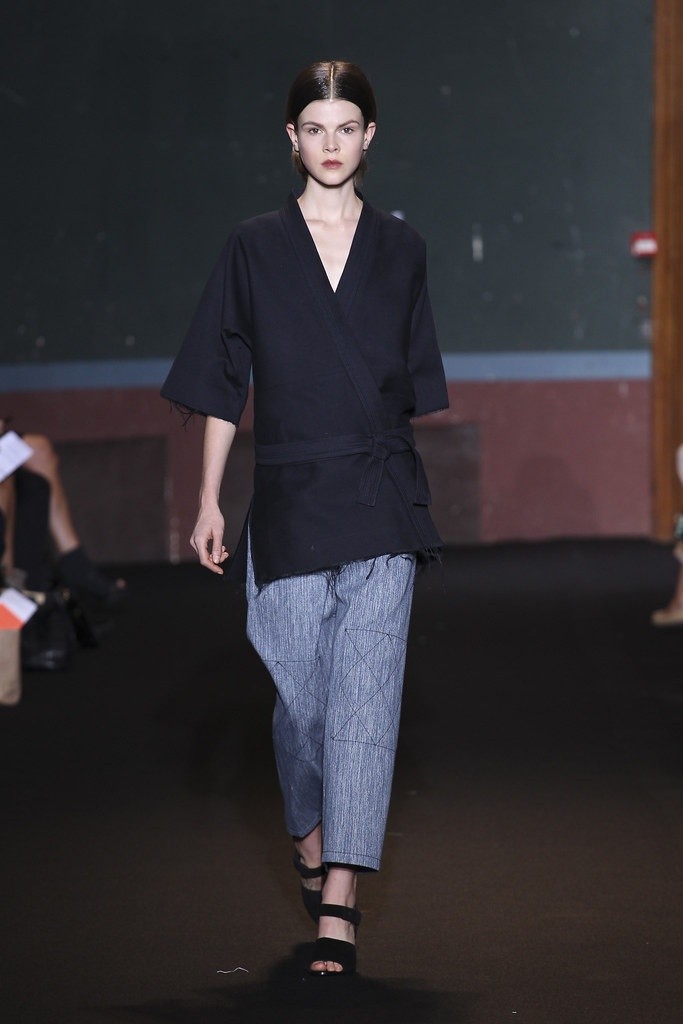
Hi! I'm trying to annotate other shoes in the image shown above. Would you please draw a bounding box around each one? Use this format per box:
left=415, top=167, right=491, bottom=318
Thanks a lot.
left=21, top=610, right=67, bottom=672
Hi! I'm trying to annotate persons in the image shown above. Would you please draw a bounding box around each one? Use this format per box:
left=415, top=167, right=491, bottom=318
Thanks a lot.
left=159, top=60, right=449, bottom=987
left=0, top=419, right=126, bottom=710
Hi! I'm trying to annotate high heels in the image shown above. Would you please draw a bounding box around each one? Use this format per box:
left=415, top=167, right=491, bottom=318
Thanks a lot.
left=312, top=904, right=360, bottom=989
left=290, top=851, right=324, bottom=922
left=61, top=550, right=126, bottom=651
